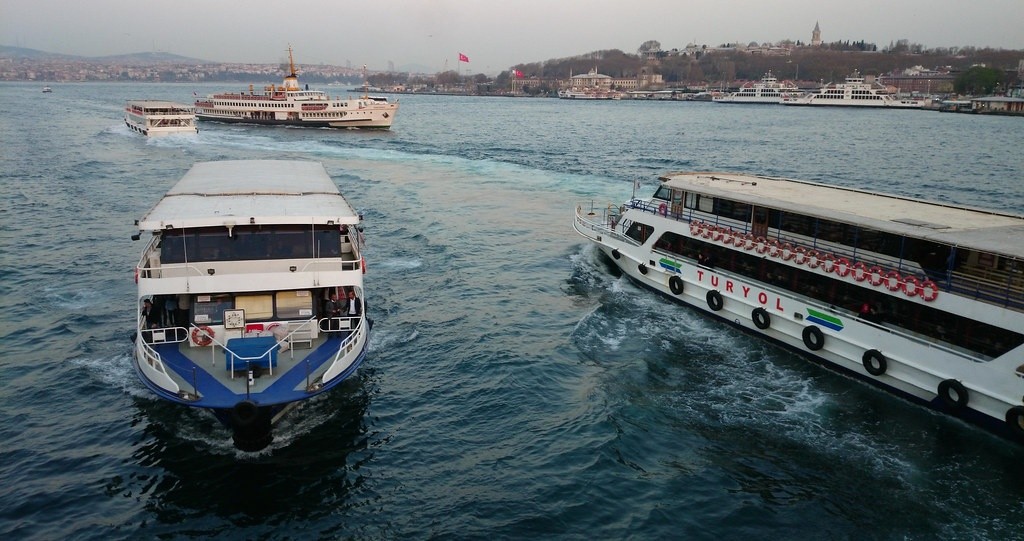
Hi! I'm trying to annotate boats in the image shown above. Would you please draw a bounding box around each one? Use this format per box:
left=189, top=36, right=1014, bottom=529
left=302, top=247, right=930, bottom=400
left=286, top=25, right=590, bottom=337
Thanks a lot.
left=711, top=69, right=806, bottom=104
left=573, top=168, right=1024, bottom=447
left=779, top=70, right=902, bottom=107
left=193, top=42, right=400, bottom=130
left=558, top=88, right=622, bottom=100
left=123, top=100, right=199, bottom=137
left=42, top=87, right=52, bottom=93
left=130, top=159, right=375, bottom=453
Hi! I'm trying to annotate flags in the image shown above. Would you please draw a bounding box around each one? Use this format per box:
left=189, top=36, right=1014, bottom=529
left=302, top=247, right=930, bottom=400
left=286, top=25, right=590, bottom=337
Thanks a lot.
left=460, top=54, right=468, bottom=62
left=512, top=68, right=523, bottom=77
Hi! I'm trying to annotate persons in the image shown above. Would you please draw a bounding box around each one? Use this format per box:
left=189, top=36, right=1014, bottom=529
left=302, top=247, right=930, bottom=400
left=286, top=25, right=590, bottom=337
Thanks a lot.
left=305, top=84, right=309, bottom=90
left=869, top=308, right=883, bottom=323
left=698, top=254, right=714, bottom=268
left=142, top=299, right=157, bottom=330
left=152, top=295, right=178, bottom=327
left=343, top=292, right=361, bottom=329
left=801, top=285, right=819, bottom=299
left=762, top=273, right=786, bottom=287
left=326, top=293, right=343, bottom=329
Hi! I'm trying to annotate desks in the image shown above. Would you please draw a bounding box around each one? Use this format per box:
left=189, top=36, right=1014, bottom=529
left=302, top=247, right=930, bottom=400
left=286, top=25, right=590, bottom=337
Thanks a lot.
left=226, top=335, right=276, bottom=370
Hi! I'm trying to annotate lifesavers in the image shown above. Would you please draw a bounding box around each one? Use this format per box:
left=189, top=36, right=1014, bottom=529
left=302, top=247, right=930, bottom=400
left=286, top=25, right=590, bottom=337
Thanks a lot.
left=266, top=322, right=280, bottom=332
left=689, top=220, right=700, bottom=235
left=669, top=276, right=684, bottom=295
left=802, top=325, right=824, bottom=350
left=192, top=326, right=214, bottom=347
left=361, top=257, right=366, bottom=274
left=133, top=266, right=139, bottom=284
left=637, top=264, right=648, bottom=275
left=752, top=307, right=771, bottom=329
left=611, top=249, right=620, bottom=260
left=1005, top=405, right=1024, bottom=437
left=657, top=204, right=668, bottom=217
left=861, top=349, right=888, bottom=377
left=701, top=223, right=939, bottom=301
left=706, top=290, right=723, bottom=310
left=937, top=379, right=969, bottom=408
